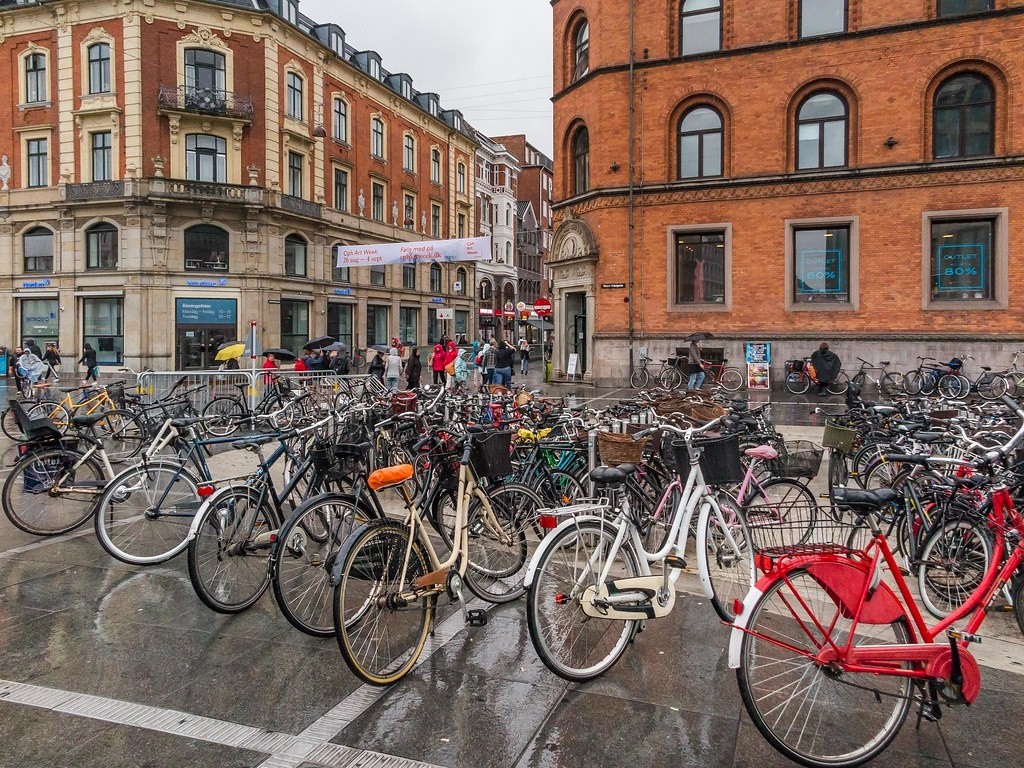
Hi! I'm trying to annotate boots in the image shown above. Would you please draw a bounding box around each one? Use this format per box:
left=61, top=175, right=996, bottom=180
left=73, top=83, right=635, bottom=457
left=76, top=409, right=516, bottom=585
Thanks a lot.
left=817, top=386, right=827, bottom=396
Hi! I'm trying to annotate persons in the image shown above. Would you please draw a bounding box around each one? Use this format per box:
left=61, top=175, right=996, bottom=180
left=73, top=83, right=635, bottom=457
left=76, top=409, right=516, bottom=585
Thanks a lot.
left=9, top=340, right=61, bottom=397
left=368, top=336, right=422, bottom=393
left=431, top=341, right=471, bottom=393
left=807, top=343, right=842, bottom=397
left=518, top=336, right=529, bottom=375
left=300, top=346, right=351, bottom=393
left=472, top=339, right=516, bottom=393
left=687, top=339, right=705, bottom=391
left=78, top=343, right=98, bottom=385
left=224, top=358, right=241, bottom=371
left=218, top=362, right=227, bottom=385
left=440, top=335, right=467, bottom=346
left=263, top=353, right=279, bottom=397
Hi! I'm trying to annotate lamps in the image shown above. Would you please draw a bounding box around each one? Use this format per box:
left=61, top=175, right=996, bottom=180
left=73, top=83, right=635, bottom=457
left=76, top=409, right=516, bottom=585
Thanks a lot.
left=312, top=123, right=327, bottom=137
left=404, top=214, right=414, bottom=225
left=498, top=258, right=504, bottom=263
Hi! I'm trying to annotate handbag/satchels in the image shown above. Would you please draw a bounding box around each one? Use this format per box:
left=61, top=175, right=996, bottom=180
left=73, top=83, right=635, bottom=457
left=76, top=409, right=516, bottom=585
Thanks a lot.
left=445, top=361, right=455, bottom=375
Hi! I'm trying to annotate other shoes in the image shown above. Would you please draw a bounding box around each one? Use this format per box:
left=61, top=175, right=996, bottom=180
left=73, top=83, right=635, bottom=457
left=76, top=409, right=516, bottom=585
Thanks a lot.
left=695, top=388, right=703, bottom=391
left=53, top=378, right=59, bottom=382
left=83, top=380, right=88, bottom=383
left=42, top=377, right=47, bottom=382
left=525, top=371, right=528, bottom=375
left=92, top=381, right=98, bottom=385
left=17, top=391, right=22, bottom=395
left=521, top=370, right=523, bottom=374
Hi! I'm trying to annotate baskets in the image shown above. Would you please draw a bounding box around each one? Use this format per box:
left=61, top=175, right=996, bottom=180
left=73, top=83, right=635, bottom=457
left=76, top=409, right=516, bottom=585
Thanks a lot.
left=668, top=358, right=676, bottom=365
left=793, top=360, right=803, bottom=371
left=691, top=400, right=727, bottom=426
left=945, top=446, right=1014, bottom=492
left=308, top=424, right=369, bottom=482
left=822, top=418, right=857, bottom=451
left=744, top=504, right=871, bottom=555
left=278, top=376, right=291, bottom=393
left=672, top=433, right=741, bottom=484
left=954, top=475, right=1024, bottom=559
left=597, top=431, right=645, bottom=465
left=773, top=440, right=825, bottom=476
left=948, top=358, right=962, bottom=370
left=142, top=406, right=189, bottom=448
left=462, top=431, right=514, bottom=477
left=392, top=392, right=418, bottom=415
left=98, top=385, right=124, bottom=405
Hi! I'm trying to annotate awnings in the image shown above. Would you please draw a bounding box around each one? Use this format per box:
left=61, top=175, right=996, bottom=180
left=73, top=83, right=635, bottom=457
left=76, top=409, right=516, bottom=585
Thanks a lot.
left=525, top=320, right=555, bottom=331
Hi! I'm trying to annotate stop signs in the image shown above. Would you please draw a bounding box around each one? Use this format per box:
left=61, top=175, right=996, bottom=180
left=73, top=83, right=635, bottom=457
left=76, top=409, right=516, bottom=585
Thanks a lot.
left=533, top=298, right=552, bottom=317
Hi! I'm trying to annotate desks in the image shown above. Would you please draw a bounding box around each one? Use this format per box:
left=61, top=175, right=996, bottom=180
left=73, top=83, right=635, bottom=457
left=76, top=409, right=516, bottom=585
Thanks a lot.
left=205, top=262, right=225, bottom=266
left=186, top=259, right=202, bottom=267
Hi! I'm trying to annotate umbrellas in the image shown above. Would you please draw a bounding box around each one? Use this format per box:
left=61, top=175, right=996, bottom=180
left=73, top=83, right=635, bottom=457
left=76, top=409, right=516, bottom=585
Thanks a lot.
left=260, top=349, right=296, bottom=369
left=214, top=341, right=247, bottom=360
left=371, top=345, right=392, bottom=362
left=302, top=336, right=348, bottom=353
left=684, top=332, right=714, bottom=347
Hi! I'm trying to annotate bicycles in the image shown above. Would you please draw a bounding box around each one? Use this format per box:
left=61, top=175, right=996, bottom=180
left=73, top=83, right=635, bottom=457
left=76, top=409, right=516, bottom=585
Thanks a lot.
left=337, top=346, right=367, bottom=368
left=0, top=351, right=1024, bottom=768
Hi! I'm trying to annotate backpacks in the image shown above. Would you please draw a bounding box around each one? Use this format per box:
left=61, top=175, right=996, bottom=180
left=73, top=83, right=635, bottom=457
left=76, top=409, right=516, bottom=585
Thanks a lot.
left=331, top=354, right=349, bottom=376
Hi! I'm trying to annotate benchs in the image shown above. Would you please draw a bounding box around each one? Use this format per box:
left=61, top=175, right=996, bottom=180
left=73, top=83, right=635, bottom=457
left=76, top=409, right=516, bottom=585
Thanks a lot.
left=182, top=377, right=248, bottom=390
